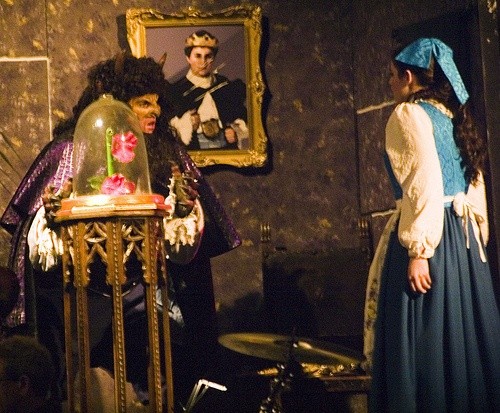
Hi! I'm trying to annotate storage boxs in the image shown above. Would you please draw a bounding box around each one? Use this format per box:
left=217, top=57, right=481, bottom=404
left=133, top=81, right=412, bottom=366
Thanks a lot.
left=67, top=92, right=163, bottom=204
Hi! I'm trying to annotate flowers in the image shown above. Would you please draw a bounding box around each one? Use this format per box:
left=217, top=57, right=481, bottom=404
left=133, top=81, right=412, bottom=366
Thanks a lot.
left=89, top=128, right=136, bottom=195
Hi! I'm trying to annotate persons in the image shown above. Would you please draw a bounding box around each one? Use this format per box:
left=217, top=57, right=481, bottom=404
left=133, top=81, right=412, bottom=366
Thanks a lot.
left=0, top=58, right=240, bottom=413
left=0, top=336, right=53, bottom=413
left=364, top=38, right=500, bottom=413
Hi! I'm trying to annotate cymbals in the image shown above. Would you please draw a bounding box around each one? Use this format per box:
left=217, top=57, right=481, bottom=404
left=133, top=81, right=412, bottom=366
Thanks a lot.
left=218, top=330, right=364, bottom=369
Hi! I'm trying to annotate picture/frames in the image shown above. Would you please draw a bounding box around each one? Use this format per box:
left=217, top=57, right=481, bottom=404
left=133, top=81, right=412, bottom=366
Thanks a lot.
left=125, top=6, right=268, bottom=170
